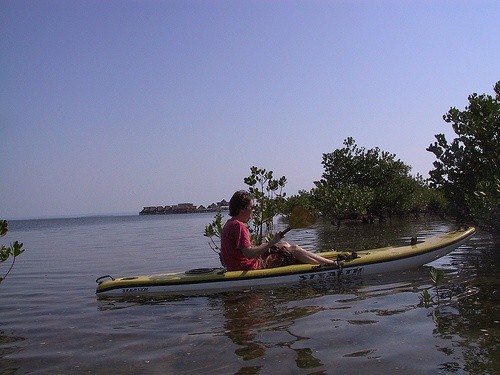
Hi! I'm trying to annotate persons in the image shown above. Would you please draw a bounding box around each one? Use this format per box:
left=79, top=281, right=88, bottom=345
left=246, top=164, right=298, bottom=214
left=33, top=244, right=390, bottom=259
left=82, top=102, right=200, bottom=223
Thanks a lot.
left=221, top=190, right=345, bottom=271
left=224, top=289, right=293, bottom=361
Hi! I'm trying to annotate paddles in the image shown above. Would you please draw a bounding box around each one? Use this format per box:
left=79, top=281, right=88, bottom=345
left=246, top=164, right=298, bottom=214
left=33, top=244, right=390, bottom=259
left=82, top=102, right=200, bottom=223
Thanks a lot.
left=263, top=205, right=317, bottom=246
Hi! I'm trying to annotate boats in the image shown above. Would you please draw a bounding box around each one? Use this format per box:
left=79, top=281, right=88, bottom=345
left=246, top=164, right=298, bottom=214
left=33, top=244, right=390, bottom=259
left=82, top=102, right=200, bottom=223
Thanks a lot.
left=95, top=224, right=477, bottom=299
